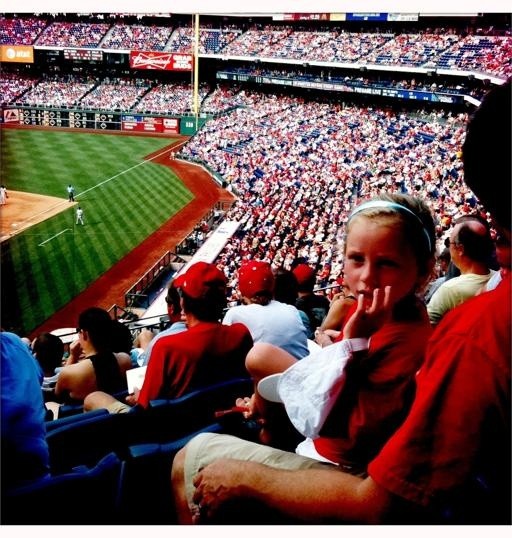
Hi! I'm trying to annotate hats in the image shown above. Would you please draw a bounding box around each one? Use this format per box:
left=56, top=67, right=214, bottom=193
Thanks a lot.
left=173, top=262, right=227, bottom=303
left=238, top=260, right=276, bottom=303
left=290, top=263, right=317, bottom=287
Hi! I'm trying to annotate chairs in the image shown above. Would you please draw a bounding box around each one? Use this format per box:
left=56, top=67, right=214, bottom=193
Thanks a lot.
left=122, top=422, right=226, bottom=515
left=45, top=410, right=110, bottom=430
left=2, top=453, right=126, bottom=526
left=46, top=405, right=142, bottom=475
left=150, top=380, right=254, bottom=425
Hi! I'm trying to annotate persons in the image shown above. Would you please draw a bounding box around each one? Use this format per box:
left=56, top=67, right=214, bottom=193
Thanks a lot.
left=0, top=328, right=52, bottom=525
left=74, top=207, right=85, bottom=227
left=171, top=81, right=511, bottom=526
left=270, top=266, right=312, bottom=342
left=64, top=184, right=77, bottom=203
left=166, top=134, right=197, bottom=163
left=0, top=14, right=210, bottom=118
left=52, top=305, right=130, bottom=403
left=224, top=257, right=313, bottom=364
left=0, top=185, right=11, bottom=206
left=290, top=263, right=330, bottom=331
left=26, top=332, right=65, bottom=403
left=196, top=12, right=511, bottom=325
left=425, top=214, right=503, bottom=331
left=108, top=318, right=131, bottom=356
left=236, top=192, right=435, bottom=481
left=128, top=274, right=189, bottom=365
left=82, top=263, right=254, bottom=416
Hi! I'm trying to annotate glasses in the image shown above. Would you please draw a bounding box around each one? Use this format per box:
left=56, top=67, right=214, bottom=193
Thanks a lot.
left=443, top=238, right=462, bottom=251
